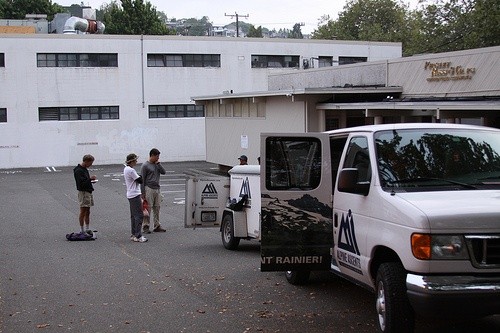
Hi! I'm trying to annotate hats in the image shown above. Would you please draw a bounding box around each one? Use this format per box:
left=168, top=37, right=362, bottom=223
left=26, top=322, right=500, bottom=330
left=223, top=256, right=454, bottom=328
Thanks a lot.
left=238, top=155, right=247, bottom=161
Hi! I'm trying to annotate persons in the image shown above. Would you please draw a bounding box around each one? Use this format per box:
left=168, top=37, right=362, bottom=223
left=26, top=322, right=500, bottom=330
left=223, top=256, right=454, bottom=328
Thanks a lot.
left=73, top=154, right=98, bottom=233
left=140, top=148, right=166, bottom=234
left=238, top=155, right=248, bottom=165
left=123, top=153, right=148, bottom=243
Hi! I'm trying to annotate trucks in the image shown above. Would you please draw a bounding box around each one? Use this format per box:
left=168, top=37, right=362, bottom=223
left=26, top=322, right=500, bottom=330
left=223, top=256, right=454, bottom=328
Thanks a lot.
left=184, top=164, right=260, bottom=251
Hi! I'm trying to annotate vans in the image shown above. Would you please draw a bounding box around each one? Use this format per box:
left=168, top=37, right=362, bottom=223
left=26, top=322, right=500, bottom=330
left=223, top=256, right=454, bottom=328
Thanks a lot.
left=260, top=122, right=500, bottom=333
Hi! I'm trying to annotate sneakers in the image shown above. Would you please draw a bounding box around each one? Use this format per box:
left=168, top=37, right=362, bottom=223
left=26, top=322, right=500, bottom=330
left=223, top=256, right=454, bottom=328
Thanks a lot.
left=130, top=234, right=145, bottom=240
left=134, top=236, right=148, bottom=242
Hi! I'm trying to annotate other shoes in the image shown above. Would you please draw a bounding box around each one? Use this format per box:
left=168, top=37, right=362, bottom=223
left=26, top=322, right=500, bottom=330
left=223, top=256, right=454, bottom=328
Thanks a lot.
left=153, top=227, right=166, bottom=232
left=143, top=226, right=151, bottom=234
